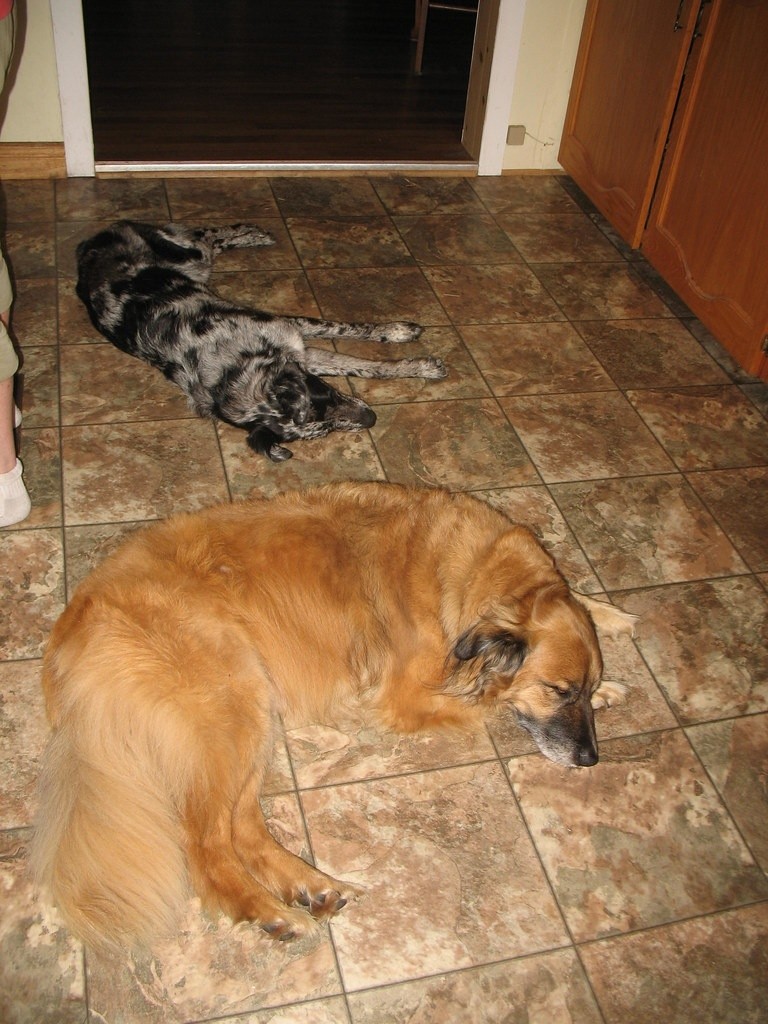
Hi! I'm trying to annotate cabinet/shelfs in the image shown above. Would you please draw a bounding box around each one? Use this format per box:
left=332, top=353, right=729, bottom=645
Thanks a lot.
left=556, top=0, right=768, bottom=387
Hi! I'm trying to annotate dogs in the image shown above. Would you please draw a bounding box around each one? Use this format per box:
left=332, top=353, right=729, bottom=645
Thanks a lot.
left=33, top=480, right=638, bottom=957
left=75, top=219, right=448, bottom=464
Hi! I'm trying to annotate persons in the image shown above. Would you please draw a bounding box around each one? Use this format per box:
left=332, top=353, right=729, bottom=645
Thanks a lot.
left=0, top=0, right=32, bottom=530
